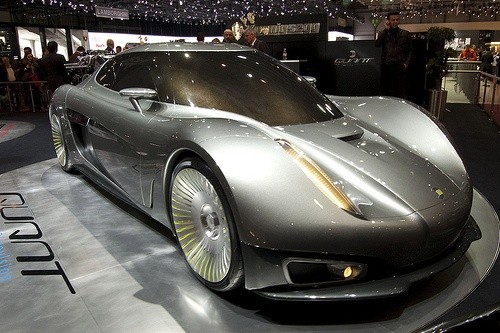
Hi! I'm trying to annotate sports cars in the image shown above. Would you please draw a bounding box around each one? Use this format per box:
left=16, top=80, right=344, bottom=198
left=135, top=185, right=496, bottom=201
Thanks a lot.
left=49, top=42, right=482, bottom=301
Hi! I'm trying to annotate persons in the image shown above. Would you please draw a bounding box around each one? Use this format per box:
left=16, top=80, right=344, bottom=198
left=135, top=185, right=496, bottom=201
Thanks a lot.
left=243, top=28, right=271, bottom=55
left=197, top=33, right=204, bottom=43
left=0, top=39, right=129, bottom=112
left=377, top=11, right=415, bottom=100
left=460, top=45, right=500, bottom=84
left=222, top=29, right=237, bottom=43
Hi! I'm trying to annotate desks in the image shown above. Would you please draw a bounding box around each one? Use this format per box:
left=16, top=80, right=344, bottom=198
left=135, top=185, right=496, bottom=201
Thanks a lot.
left=0, top=81, right=47, bottom=113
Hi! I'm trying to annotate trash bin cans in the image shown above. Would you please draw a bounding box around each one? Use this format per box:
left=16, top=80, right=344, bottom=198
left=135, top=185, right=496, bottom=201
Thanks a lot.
left=426, top=89, right=447, bottom=123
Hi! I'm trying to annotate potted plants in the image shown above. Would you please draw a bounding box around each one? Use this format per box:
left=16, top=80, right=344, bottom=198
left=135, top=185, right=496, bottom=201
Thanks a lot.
left=423, top=27, right=462, bottom=122
